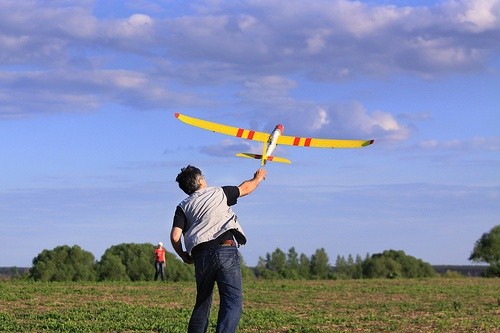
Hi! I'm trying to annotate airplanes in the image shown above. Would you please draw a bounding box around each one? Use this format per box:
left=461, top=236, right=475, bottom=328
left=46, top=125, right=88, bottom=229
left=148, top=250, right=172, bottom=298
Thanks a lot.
left=173, top=111, right=375, bottom=167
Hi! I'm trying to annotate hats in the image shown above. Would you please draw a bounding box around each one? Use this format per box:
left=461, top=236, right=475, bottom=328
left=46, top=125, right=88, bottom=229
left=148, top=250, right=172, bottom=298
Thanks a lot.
left=158, top=242, right=163, bottom=246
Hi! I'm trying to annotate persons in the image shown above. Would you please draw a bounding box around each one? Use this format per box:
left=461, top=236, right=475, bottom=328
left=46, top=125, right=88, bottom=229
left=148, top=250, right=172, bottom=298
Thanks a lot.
left=170, top=165, right=268, bottom=333
left=154, top=242, right=166, bottom=283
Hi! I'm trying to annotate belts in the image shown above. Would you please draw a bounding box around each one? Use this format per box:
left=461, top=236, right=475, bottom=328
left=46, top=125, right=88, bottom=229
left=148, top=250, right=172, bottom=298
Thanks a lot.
left=223, top=240, right=236, bottom=246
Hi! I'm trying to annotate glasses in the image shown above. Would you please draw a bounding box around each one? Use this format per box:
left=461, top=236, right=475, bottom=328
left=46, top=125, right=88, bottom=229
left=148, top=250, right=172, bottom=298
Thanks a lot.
left=198, top=174, right=205, bottom=181
